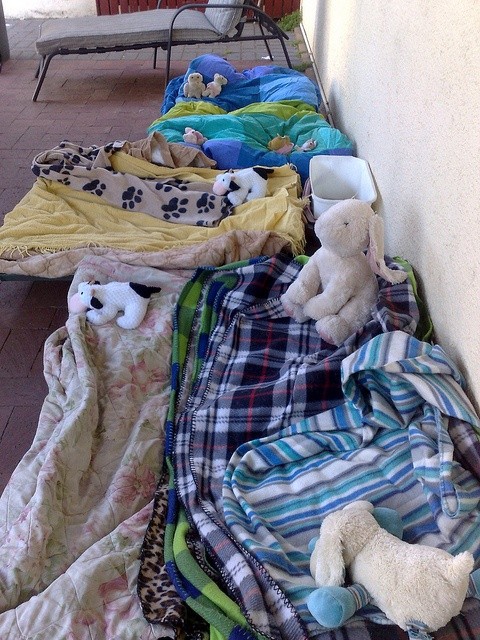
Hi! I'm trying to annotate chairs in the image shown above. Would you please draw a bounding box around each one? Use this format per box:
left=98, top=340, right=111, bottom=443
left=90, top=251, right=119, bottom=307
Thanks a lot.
left=32, top=1, right=293, bottom=102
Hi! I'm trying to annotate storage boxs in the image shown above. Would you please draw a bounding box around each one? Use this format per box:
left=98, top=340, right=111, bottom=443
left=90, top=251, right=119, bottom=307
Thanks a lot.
left=309, top=154, right=378, bottom=220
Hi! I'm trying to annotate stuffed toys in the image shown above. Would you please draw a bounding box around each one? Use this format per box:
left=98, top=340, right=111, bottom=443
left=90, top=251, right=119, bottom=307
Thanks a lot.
left=67, top=278, right=161, bottom=329
left=201, top=73, right=228, bottom=98
left=306, top=500, right=475, bottom=640
left=183, top=73, right=206, bottom=98
left=183, top=126, right=208, bottom=145
left=301, top=139, right=317, bottom=151
left=212, top=167, right=274, bottom=206
left=267, top=136, right=294, bottom=153
left=279, top=197, right=408, bottom=347
